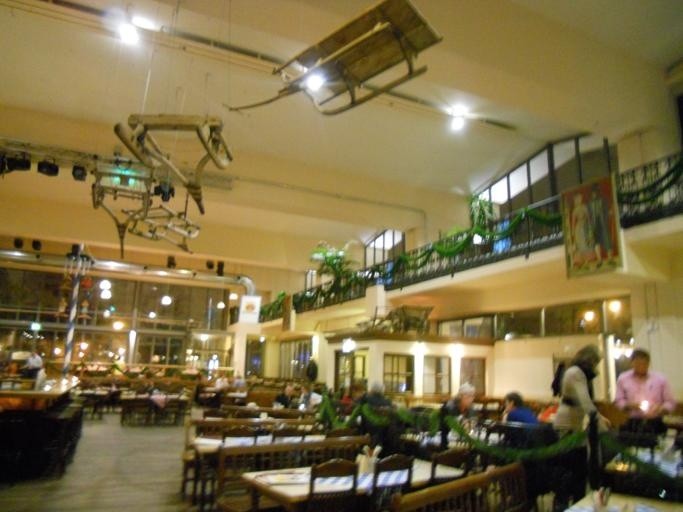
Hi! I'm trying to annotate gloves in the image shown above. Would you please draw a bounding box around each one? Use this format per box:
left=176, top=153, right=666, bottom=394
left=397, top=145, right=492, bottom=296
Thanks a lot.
left=594, top=411, right=612, bottom=434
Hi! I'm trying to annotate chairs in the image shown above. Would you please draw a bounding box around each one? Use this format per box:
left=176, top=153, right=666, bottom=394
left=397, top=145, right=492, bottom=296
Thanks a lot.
left=83, top=378, right=198, bottom=427
left=184, top=377, right=532, bottom=512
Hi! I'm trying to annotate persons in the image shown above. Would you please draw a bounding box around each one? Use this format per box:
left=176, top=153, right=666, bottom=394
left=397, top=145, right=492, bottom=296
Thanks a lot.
left=553, top=345, right=612, bottom=512
left=440, top=383, right=476, bottom=450
left=275, top=383, right=324, bottom=409
left=207, top=354, right=219, bottom=381
left=351, top=380, right=392, bottom=434
left=615, top=348, right=675, bottom=448
left=571, top=188, right=616, bottom=271
left=23, top=351, right=43, bottom=379
left=502, top=392, right=538, bottom=424
left=215, top=374, right=246, bottom=389
